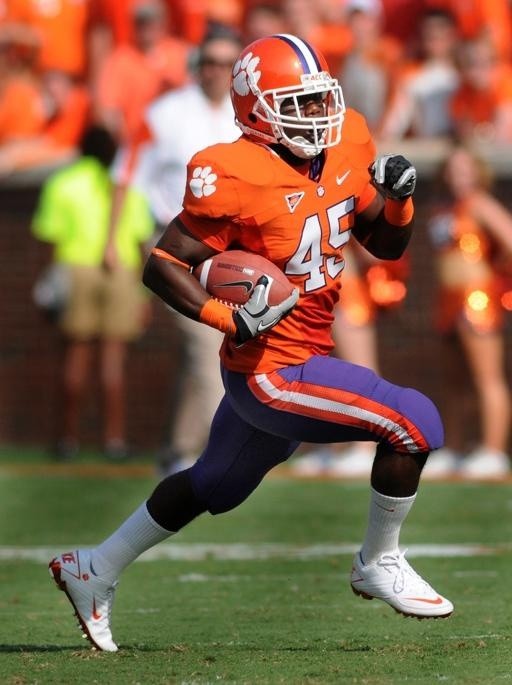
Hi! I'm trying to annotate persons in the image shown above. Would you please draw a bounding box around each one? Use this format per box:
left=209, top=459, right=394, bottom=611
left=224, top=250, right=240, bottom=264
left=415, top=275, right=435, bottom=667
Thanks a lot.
left=2, top=1, right=509, bottom=474
left=50, top=31, right=453, bottom=655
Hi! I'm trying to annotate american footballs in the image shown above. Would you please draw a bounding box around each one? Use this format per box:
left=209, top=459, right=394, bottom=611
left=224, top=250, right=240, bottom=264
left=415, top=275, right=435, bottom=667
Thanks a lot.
left=192, top=252, right=293, bottom=312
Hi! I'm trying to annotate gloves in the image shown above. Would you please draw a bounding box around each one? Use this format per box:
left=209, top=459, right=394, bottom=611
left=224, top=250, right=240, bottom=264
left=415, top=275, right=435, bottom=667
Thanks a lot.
left=369, top=151, right=417, bottom=200
left=233, top=276, right=300, bottom=344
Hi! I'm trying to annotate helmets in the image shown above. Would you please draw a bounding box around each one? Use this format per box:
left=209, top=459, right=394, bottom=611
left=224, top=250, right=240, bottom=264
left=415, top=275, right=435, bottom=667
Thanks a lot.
left=230, top=33, right=346, bottom=159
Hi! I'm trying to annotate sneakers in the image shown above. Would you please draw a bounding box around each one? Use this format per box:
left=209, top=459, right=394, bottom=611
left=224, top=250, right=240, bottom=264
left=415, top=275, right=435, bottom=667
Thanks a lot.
left=48, top=548, right=119, bottom=651
left=350, top=549, right=454, bottom=619
